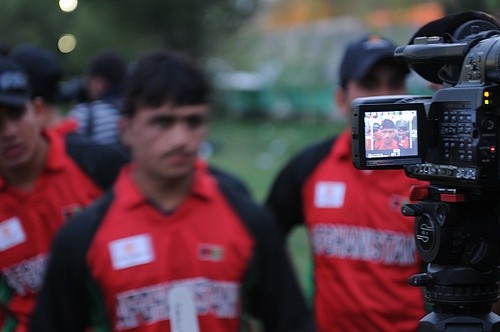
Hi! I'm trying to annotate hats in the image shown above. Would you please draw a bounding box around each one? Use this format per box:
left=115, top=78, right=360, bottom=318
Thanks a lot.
left=368, top=123, right=381, bottom=133
left=340, top=35, right=411, bottom=80
left=0, top=58, right=39, bottom=104
left=11, top=48, right=71, bottom=101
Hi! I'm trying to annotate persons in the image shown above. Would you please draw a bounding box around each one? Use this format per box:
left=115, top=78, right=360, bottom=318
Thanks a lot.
left=377, top=118, right=403, bottom=149
left=397, top=126, right=409, bottom=148
left=262, top=33, right=431, bottom=331
left=1, top=45, right=76, bottom=134
left=31, top=52, right=317, bottom=331
left=0, top=67, right=130, bottom=331
left=367, top=123, right=382, bottom=149
left=65, top=56, right=132, bottom=172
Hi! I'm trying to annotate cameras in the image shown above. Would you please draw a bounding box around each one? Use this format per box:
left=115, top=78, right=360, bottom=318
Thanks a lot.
left=349, top=11, right=500, bottom=332
